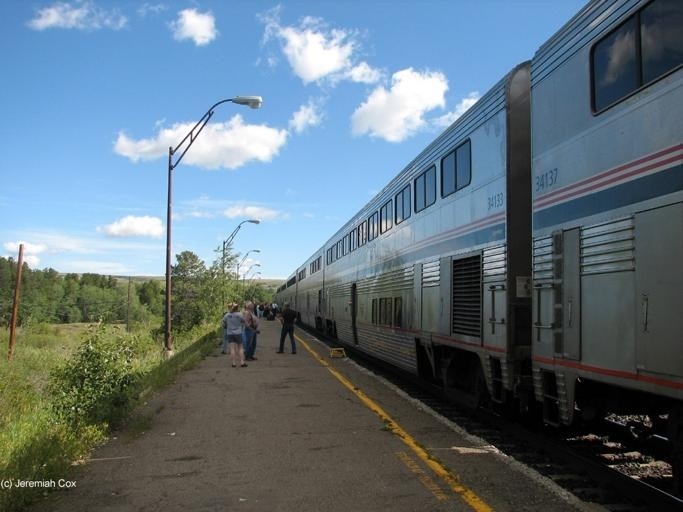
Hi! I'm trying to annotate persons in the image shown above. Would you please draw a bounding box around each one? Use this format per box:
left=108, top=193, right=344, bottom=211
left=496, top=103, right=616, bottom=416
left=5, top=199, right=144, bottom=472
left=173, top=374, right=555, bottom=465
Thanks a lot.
left=274, top=301, right=298, bottom=354
left=219, top=297, right=279, bottom=362
left=221, top=303, right=250, bottom=368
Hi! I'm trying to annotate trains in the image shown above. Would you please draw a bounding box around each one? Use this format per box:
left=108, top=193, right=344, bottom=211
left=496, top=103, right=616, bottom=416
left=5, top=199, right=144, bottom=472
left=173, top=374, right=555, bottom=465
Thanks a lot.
left=272, top=0, right=682, bottom=434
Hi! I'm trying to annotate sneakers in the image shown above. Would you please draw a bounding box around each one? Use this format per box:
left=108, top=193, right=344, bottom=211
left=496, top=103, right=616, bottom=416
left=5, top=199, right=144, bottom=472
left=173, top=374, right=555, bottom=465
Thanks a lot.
left=220, top=349, right=296, bottom=368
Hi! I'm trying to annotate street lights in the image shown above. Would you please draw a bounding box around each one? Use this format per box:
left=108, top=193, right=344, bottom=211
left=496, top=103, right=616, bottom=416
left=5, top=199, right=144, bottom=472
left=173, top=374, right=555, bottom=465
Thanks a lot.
left=163, top=95, right=262, bottom=349
